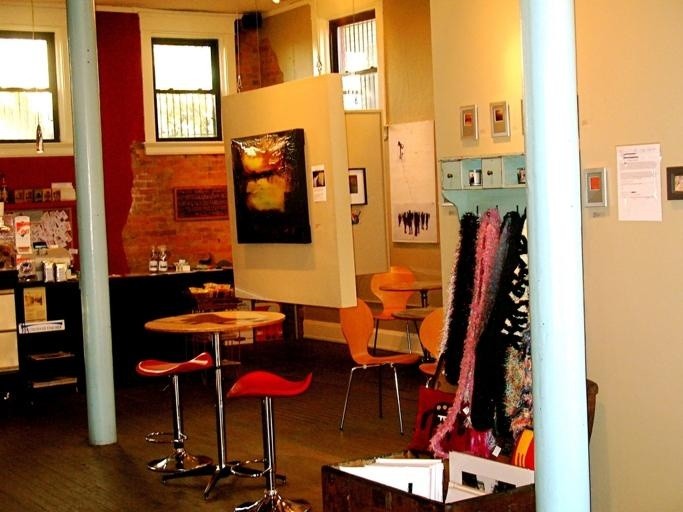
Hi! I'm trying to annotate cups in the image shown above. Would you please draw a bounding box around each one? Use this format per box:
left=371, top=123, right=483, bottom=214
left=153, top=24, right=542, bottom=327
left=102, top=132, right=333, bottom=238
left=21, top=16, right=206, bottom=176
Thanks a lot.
left=469, top=168, right=480, bottom=185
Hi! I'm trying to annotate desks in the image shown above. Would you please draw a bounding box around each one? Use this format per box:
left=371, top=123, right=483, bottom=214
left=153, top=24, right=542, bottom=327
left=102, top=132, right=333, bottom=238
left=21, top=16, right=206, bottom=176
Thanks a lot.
left=389, top=305, right=433, bottom=358
left=143, top=307, right=288, bottom=501
left=379, top=281, right=445, bottom=307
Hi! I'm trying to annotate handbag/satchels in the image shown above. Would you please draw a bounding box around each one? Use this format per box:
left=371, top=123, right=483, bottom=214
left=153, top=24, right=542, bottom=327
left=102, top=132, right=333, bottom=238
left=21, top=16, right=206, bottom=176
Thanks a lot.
left=408, top=352, right=469, bottom=454
left=511, top=428, right=534, bottom=470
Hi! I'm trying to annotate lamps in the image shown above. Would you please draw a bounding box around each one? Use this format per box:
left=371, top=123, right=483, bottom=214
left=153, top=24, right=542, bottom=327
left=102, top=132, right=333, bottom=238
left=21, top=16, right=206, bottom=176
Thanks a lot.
left=29, top=1, right=45, bottom=157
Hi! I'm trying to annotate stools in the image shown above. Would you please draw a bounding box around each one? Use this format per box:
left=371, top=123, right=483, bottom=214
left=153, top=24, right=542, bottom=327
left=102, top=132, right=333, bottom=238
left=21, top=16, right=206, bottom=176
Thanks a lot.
left=226, top=367, right=312, bottom=510
left=133, top=351, right=213, bottom=480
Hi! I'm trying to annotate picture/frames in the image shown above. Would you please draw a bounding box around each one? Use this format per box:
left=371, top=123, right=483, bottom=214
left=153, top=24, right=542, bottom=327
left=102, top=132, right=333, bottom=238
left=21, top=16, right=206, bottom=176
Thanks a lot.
left=583, top=167, right=608, bottom=209
left=347, top=166, right=368, bottom=207
left=666, top=165, right=683, bottom=200
left=489, top=100, right=511, bottom=138
left=456, top=104, right=479, bottom=141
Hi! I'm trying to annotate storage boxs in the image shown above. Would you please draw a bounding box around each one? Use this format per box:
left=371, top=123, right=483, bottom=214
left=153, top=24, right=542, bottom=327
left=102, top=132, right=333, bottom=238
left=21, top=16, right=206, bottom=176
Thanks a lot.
left=317, top=455, right=535, bottom=511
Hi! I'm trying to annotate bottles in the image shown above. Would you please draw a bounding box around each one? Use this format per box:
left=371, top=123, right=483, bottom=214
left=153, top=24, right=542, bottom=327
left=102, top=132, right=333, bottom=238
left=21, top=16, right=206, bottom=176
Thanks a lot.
left=516, top=167, right=525, bottom=183
left=148, top=246, right=158, bottom=272
left=156, top=246, right=167, bottom=272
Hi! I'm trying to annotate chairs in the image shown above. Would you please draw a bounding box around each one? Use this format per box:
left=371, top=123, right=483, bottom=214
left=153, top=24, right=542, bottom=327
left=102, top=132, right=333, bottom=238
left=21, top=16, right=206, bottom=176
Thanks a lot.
left=364, top=269, right=419, bottom=357
left=418, top=305, right=445, bottom=394
left=336, top=296, right=421, bottom=435
